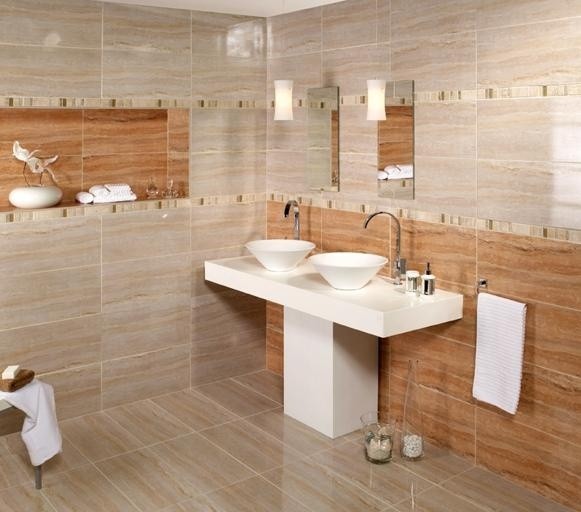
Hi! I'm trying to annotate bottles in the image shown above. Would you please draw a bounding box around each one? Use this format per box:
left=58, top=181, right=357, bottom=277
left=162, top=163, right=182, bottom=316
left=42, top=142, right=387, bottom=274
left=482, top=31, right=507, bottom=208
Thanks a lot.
left=145, top=175, right=159, bottom=200
left=399, top=357, right=424, bottom=460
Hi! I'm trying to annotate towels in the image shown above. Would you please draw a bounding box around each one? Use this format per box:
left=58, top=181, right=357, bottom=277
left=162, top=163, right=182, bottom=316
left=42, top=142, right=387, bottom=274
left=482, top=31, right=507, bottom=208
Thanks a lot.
left=76, top=183, right=137, bottom=204
left=472, top=292, right=527, bottom=415
left=377, top=164, right=414, bottom=180
left=0, top=379, right=63, bottom=466
left=0, top=367, right=35, bottom=392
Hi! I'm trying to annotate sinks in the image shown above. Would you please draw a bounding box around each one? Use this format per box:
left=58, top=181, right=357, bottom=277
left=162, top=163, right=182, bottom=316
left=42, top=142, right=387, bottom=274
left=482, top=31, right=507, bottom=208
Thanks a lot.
left=245, top=239, right=316, bottom=271
left=309, top=252, right=389, bottom=290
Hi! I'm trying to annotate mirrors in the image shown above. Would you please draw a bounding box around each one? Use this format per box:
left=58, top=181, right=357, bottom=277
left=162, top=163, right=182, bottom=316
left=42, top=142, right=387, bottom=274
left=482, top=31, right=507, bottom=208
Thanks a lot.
left=307, top=87, right=339, bottom=192
left=378, top=80, right=414, bottom=199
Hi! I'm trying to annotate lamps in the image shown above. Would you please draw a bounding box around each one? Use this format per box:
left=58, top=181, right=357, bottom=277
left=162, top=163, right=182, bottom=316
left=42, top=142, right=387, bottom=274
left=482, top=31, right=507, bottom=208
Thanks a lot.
left=274, top=80, right=293, bottom=120
left=366, top=80, right=387, bottom=120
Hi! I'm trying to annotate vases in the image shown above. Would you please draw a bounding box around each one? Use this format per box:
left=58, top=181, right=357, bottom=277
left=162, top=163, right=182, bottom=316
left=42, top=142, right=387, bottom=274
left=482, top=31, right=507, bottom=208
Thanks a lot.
left=401, top=360, right=425, bottom=462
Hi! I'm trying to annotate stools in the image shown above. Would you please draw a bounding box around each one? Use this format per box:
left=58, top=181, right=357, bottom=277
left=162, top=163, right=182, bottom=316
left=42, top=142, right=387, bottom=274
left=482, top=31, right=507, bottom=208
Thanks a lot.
left=0, top=379, right=52, bottom=490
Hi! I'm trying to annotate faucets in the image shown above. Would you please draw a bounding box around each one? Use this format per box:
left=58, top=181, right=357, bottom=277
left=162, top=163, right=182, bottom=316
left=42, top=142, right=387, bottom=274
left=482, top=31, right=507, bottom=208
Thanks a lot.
left=283, top=199, right=300, bottom=240
left=362, top=210, right=402, bottom=287
